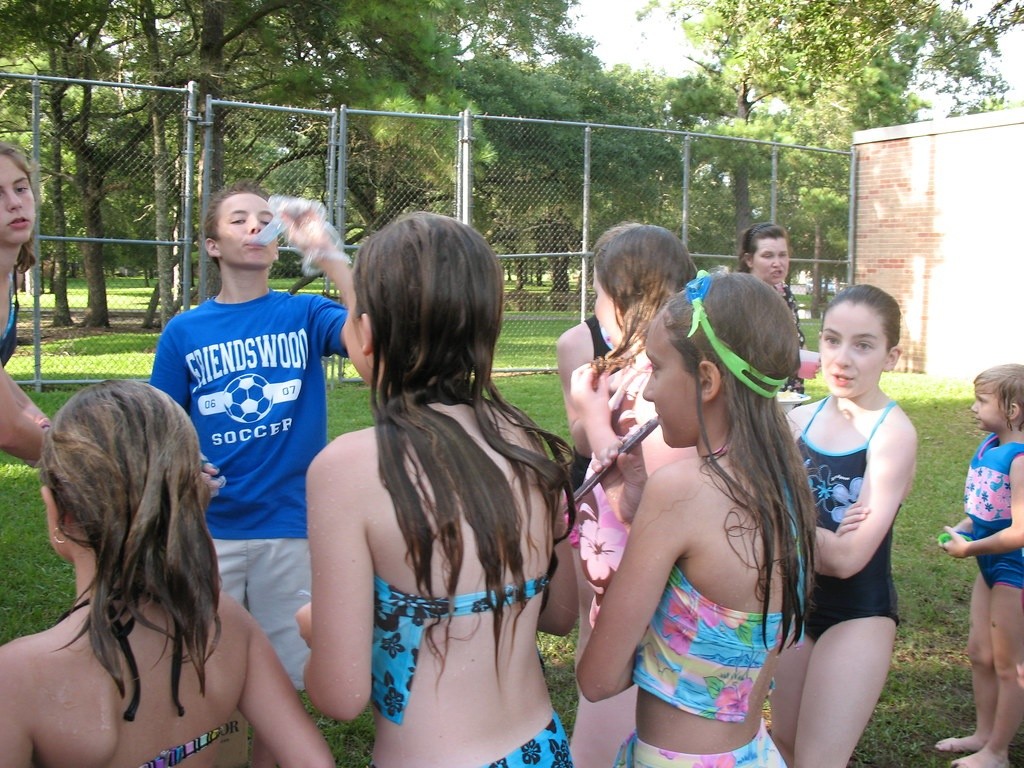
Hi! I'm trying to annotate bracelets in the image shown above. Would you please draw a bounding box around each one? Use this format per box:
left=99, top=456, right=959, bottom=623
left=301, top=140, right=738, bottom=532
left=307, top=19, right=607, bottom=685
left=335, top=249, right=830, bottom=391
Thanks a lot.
left=36, top=416, right=51, bottom=430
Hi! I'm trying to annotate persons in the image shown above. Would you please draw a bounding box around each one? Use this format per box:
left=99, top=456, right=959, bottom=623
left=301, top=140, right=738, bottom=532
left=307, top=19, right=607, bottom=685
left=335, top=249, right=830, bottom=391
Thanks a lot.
left=149, top=179, right=353, bottom=768
left=0, top=378, right=336, bottom=768
left=935, top=364, right=1024, bottom=768
left=0, top=141, right=222, bottom=499
left=557, top=220, right=918, bottom=768
left=294, top=213, right=578, bottom=768
